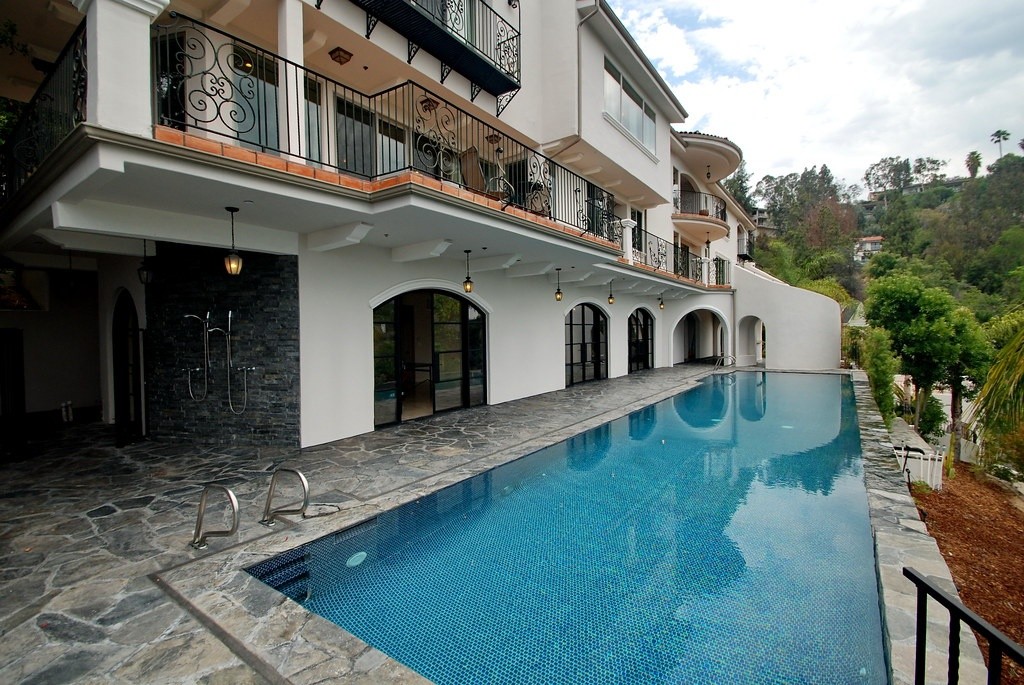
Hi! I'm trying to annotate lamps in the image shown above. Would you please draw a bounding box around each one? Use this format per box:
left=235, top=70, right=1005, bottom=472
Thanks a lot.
left=464, top=250, right=474, bottom=293
left=704, top=232, right=711, bottom=249
left=328, top=46, right=353, bottom=66
left=658, top=293, right=664, bottom=310
left=555, top=268, right=563, bottom=301
left=420, top=98, right=439, bottom=110
left=706, top=165, right=711, bottom=179
left=223, top=206, right=243, bottom=276
left=608, top=281, right=615, bottom=304
left=137, top=239, right=152, bottom=284
left=485, top=134, right=501, bottom=144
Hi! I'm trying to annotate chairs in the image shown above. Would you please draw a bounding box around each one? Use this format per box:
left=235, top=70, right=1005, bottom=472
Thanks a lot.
left=458, top=145, right=506, bottom=202
